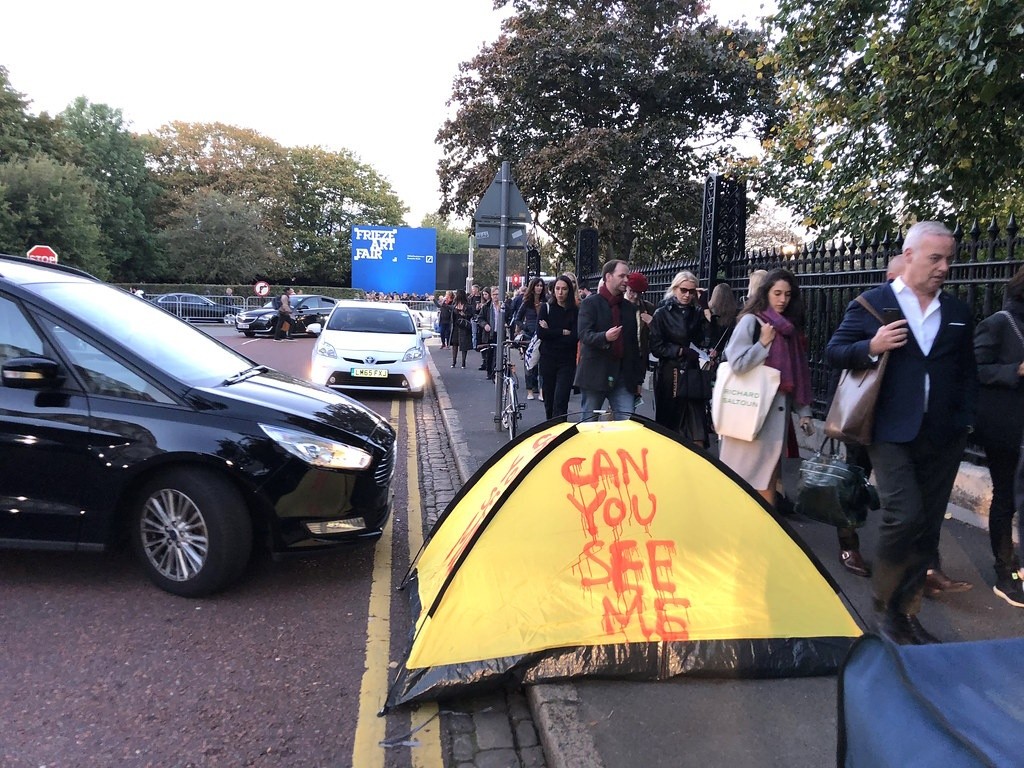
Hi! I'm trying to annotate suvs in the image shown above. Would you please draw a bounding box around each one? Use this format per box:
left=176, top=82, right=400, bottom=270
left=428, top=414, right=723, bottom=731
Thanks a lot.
left=0, top=254, right=398, bottom=598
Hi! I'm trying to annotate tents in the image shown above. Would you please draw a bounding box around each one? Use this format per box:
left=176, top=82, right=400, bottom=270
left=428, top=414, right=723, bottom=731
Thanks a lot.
left=375, top=408, right=869, bottom=718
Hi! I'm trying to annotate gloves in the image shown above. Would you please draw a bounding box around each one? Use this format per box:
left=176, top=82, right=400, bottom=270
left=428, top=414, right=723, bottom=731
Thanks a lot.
left=682, top=347, right=699, bottom=361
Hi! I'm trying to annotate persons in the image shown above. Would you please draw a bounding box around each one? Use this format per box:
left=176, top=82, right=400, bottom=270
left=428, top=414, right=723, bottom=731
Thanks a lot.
left=683, top=278, right=739, bottom=449
left=535, top=272, right=593, bottom=421
left=354, top=290, right=438, bottom=310
left=717, top=267, right=815, bottom=510
left=479, top=277, right=548, bottom=401
left=273, top=286, right=296, bottom=342
left=650, top=270, right=703, bottom=437
left=973, top=268, right=1024, bottom=607
left=825, top=220, right=982, bottom=646
left=437, top=284, right=481, bottom=368
left=291, top=289, right=303, bottom=294
left=574, top=259, right=655, bottom=420
left=224, top=288, right=236, bottom=305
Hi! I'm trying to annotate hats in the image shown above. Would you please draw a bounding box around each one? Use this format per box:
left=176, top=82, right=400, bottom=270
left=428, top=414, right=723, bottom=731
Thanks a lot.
left=627, top=272, right=648, bottom=292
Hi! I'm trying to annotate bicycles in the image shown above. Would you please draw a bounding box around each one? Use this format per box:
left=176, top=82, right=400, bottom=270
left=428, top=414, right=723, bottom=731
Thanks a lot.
left=478, top=340, right=532, bottom=441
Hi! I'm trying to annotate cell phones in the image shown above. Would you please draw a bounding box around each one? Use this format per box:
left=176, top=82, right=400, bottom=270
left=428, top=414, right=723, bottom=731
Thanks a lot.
left=882, top=307, right=900, bottom=327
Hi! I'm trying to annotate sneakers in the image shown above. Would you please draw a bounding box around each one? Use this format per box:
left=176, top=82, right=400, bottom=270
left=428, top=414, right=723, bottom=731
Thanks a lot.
left=994, top=579, right=1024, bottom=607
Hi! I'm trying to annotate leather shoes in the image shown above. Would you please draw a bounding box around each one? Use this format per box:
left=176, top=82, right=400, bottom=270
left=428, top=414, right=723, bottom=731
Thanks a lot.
left=884, top=609, right=942, bottom=645
left=839, top=549, right=871, bottom=576
left=924, top=568, right=972, bottom=598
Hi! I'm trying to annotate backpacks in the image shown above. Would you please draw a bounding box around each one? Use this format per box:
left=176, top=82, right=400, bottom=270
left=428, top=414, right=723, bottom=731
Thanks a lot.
left=272, top=295, right=283, bottom=310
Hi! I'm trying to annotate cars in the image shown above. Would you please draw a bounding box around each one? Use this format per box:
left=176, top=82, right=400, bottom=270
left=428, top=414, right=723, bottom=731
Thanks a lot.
left=409, top=303, right=441, bottom=328
left=306, top=301, right=433, bottom=400
left=148, top=293, right=243, bottom=325
left=234, top=295, right=336, bottom=337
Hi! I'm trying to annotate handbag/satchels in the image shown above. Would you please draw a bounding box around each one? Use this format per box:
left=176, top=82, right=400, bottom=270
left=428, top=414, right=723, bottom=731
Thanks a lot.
left=795, top=431, right=880, bottom=529
left=672, top=360, right=712, bottom=399
left=698, top=357, right=710, bottom=370
left=524, top=302, right=552, bottom=371
left=509, top=300, right=523, bottom=349
left=457, top=312, right=472, bottom=330
left=711, top=316, right=781, bottom=442
left=824, top=295, right=891, bottom=445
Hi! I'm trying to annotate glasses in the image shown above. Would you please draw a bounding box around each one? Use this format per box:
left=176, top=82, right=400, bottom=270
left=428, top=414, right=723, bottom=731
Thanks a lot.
left=676, top=286, right=695, bottom=294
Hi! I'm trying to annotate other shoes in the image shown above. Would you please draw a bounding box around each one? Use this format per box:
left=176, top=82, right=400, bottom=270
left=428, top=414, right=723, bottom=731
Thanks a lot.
left=479, top=363, right=486, bottom=370
left=440, top=344, right=445, bottom=349
left=462, top=364, right=465, bottom=369
left=486, top=374, right=494, bottom=380
left=451, top=363, right=455, bottom=368
left=527, top=393, right=534, bottom=400
left=776, top=491, right=797, bottom=515
left=447, top=344, right=450, bottom=349
left=538, top=396, right=544, bottom=401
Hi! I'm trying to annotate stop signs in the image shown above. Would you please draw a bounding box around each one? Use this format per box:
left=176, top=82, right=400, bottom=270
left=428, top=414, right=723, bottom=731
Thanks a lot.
left=27, top=246, right=58, bottom=263
